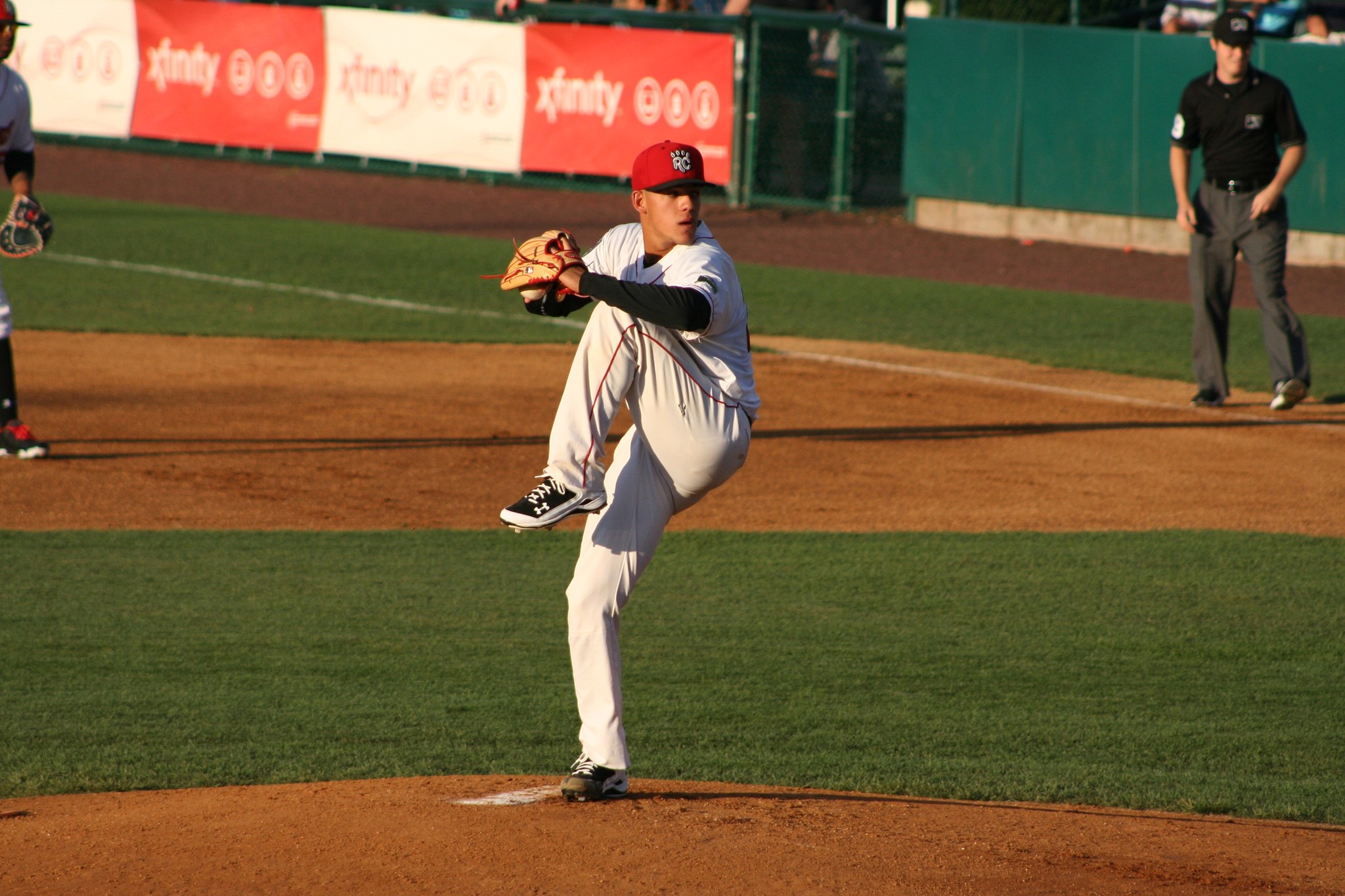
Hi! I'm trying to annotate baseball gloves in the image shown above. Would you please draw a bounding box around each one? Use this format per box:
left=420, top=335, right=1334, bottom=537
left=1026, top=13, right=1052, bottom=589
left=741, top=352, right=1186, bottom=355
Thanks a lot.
left=478, top=227, right=592, bottom=300
left=0, top=192, right=53, bottom=259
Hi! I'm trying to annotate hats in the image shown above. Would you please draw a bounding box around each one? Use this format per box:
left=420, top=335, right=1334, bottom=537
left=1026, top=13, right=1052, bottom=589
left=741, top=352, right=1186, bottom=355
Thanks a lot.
left=631, top=140, right=716, bottom=191
left=0, top=0, right=30, bottom=26
left=1213, top=12, right=1256, bottom=45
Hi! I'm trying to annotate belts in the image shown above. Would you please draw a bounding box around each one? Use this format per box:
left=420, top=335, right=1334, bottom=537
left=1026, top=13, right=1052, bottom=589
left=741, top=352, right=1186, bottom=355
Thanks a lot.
left=1205, top=177, right=1270, bottom=193
left=741, top=408, right=752, bottom=429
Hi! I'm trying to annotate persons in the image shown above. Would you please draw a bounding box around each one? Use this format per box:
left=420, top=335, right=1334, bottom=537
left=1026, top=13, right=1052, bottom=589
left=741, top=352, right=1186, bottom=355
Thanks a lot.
left=1160, top=0, right=1345, bottom=47
left=499, top=140, right=760, bottom=801
left=0, top=0, right=56, bottom=459
left=1170, top=10, right=1312, bottom=410
left=492, top=0, right=932, bottom=149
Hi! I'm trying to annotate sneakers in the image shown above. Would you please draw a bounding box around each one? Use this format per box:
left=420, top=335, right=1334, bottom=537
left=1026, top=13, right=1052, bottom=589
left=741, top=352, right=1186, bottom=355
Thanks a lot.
left=1189, top=387, right=1225, bottom=406
left=1270, top=377, right=1308, bottom=410
left=500, top=473, right=607, bottom=533
left=0, top=419, right=49, bottom=459
left=560, top=752, right=628, bottom=801
